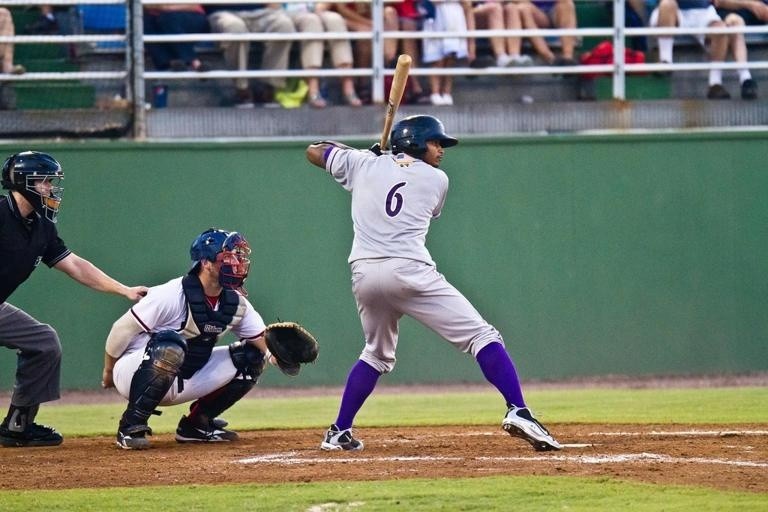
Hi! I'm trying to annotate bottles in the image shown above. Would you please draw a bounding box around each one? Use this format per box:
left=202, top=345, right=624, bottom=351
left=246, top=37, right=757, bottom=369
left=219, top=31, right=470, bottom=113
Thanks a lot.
left=319, top=77, right=328, bottom=103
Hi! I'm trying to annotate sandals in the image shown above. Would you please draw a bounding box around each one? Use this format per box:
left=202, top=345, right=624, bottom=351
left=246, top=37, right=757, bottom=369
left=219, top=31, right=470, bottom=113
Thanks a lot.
left=306, top=92, right=328, bottom=109
left=342, top=92, right=363, bottom=107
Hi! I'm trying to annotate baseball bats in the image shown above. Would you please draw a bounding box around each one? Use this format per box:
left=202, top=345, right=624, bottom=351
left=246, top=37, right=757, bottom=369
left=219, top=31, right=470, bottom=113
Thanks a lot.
left=381, top=55, right=413, bottom=151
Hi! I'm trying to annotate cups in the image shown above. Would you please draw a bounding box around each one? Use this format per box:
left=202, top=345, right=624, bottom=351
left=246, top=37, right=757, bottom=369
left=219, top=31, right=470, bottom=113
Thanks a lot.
left=152, top=83, right=168, bottom=108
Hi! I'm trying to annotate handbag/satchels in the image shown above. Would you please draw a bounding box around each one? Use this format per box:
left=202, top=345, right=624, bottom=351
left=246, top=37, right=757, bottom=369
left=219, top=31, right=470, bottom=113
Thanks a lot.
left=579, top=40, right=646, bottom=77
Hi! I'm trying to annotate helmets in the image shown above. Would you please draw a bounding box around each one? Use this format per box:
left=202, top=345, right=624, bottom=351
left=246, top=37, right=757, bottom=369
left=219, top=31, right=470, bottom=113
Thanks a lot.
left=0, top=151, right=64, bottom=224
left=390, top=114, right=458, bottom=155
left=188, top=227, right=252, bottom=297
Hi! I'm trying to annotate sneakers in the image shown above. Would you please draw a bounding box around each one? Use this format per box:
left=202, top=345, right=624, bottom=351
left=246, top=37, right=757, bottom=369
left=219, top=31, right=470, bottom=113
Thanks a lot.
left=175, top=415, right=239, bottom=442
left=429, top=92, right=455, bottom=107
left=494, top=53, right=532, bottom=67
left=320, top=424, right=364, bottom=452
left=232, top=85, right=281, bottom=109
left=706, top=78, right=759, bottom=100
left=0, top=423, right=63, bottom=447
left=502, top=407, right=564, bottom=451
left=116, top=420, right=152, bottom=450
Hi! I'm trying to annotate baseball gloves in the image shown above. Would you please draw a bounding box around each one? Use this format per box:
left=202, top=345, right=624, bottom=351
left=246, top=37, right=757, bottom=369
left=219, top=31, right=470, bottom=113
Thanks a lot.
left=264, top=323, right=319, bottom=377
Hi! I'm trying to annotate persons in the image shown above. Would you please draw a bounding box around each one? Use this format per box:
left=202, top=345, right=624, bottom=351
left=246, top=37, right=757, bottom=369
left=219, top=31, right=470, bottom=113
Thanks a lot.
left=0, top=150, right=148, bottom=446
left=0, top=5, right=69, bottom=74
left=303, top=114, right=563, bottom=451
left=101, top=227, right=319, bottom=450
left=144, top=1, right=768, bottom=110
